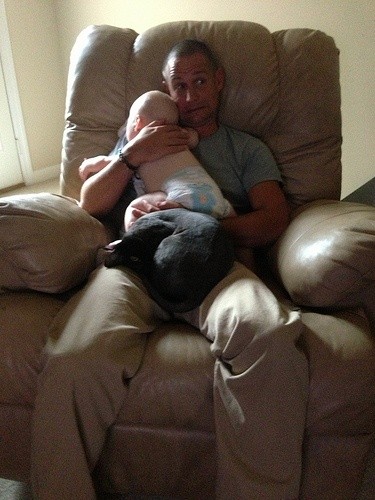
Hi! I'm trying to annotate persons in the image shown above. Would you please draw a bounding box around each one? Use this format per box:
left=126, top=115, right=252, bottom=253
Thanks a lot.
left=78, top=91, right=237, bottom=234
left=31, top=39, right=309, bottom=499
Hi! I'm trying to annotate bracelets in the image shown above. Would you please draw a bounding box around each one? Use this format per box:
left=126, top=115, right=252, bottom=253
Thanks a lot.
left=118, top=149, right=141, bottom=179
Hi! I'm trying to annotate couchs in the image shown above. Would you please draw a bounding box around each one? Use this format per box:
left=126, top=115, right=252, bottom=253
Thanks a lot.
left=0, top=21, right=375, bottom=500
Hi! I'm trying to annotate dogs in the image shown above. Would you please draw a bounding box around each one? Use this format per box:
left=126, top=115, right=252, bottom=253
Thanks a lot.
left=101, top=206, right=237, bottom=305
